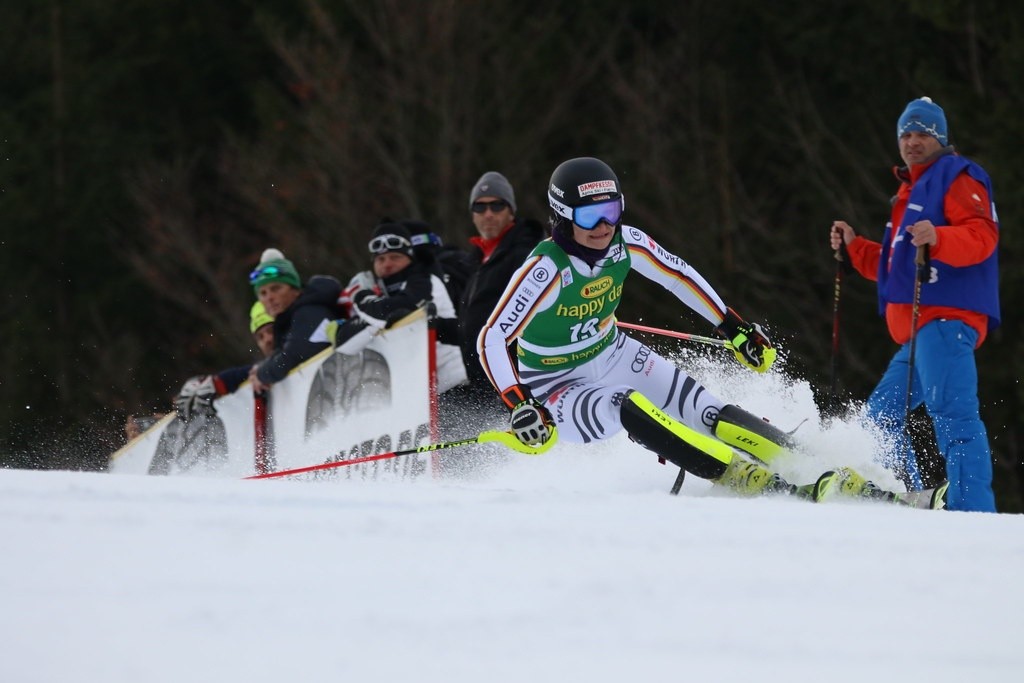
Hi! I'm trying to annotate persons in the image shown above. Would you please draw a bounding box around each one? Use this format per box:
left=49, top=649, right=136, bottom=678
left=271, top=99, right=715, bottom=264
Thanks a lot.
left=831, top=97, right=1000, bottom=513
left=171, top=215, right=475, bottom=423
left=385, top=172, right=544, bottom=412
left=476, top=158, right=861, bottom=502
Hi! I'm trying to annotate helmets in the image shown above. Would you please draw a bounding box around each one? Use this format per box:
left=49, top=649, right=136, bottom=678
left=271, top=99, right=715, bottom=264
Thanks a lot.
left=548, top=157, right=625, bottom=240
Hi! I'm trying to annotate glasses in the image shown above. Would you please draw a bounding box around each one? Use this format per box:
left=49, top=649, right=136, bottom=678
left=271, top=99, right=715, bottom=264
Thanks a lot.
left=368, top=233, right=411, bottom=254
left=572, top=197, right=623, bottom=230
left=472, top=200, right=507, bottom=213
left=248, top=265, right=290, bottom=285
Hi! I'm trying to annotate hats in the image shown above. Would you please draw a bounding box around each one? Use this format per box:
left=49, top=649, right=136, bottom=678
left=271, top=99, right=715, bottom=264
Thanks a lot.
left=370, top=218, right=414, bottom=259
left=896, top=96, right=947, bottom=146
left=249, top=301, right=276, bottom=334
left=469, top=171, right=517, bottom=216
left=252, top=248, right=300, bottom=298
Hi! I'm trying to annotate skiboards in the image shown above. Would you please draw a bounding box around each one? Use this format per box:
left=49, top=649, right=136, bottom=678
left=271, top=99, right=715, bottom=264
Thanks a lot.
left=835, top=467, right=950, bottom=509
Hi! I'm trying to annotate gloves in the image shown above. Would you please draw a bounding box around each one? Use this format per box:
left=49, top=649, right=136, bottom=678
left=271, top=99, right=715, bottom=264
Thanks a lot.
left=192, top=375, right=217, bottom=417
left=350, top=271, right=374, bottom=299
left=502, top=386, right=555, bottom=447
left=175, top=378, right=199, bottom=418
left=717, top=306, right=771, bottom=367
